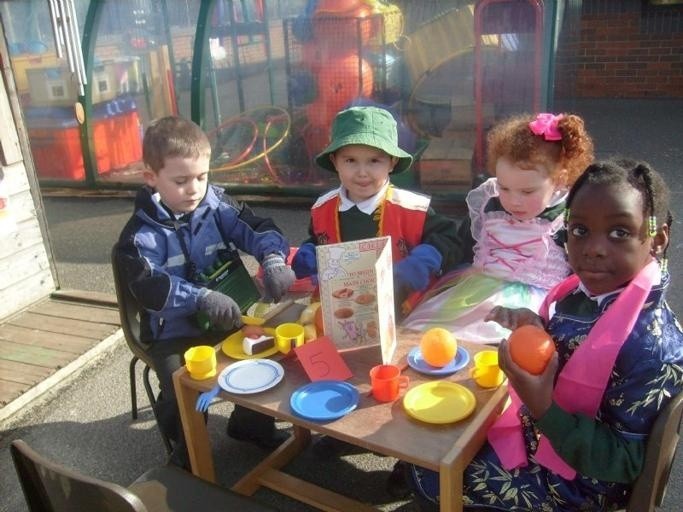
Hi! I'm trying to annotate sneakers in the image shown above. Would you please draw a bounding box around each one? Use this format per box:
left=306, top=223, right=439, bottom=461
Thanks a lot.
left=226, top=410, right=292, bottom=451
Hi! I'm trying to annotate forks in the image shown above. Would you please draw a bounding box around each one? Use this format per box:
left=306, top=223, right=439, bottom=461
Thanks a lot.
left=193, top=385, right=218, bottom=413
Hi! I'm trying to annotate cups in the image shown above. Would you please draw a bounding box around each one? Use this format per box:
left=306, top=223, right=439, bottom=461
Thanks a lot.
left=277, top=322, right=306, bottom=353
left=184, top=344, right=216, bottom=380
left=474, top=350, right=502, bottom=387
left=369, top=364, right=408, bottom=402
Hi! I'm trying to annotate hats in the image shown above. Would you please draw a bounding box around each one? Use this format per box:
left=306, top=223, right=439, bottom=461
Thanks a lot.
left=315, top=105, right=414, bottom=176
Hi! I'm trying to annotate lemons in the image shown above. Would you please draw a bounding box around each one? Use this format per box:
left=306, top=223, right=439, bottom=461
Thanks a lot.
left=421, top=328, right=457, bottom=368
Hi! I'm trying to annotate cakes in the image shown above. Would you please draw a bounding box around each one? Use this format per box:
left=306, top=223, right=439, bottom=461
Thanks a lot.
left=242, top=327, right=274, bottom=355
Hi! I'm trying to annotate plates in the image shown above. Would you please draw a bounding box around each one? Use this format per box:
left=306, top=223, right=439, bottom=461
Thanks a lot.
left=221, top=326, right=280, bottom=360
left=218, top=359, right=284, bottom=394
left=401, top=381, right=476, bottom=426
left=407, top=343, right=470, bottom=376
left=288, top=380, right=359, bottom=421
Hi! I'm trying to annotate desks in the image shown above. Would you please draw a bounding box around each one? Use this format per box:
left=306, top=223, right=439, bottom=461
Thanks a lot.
left=172, top=300, right=511, bottom=512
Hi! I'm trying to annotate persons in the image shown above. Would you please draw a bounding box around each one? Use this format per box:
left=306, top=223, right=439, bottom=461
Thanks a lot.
left=293, top=108, right=458, bottom=322
left=384, top=156, right=683, bottom=511
left=398, top=111, right=594, bottom=351
left=115, top=115, right=297, bottom=472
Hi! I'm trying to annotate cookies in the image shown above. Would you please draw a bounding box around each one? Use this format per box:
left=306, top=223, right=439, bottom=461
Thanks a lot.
left=332, top=288, right=353, bottom=299
left=334, top=308, right=353, bottom=319
left=356, top=294, right=375, bottom=304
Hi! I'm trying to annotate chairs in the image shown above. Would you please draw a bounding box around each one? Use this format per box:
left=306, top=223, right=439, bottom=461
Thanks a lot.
left=11, top=438, right=274, bottom=511
left=633, top=391, right=683, bottom=512
left=112, top=241, right=174, bottom=456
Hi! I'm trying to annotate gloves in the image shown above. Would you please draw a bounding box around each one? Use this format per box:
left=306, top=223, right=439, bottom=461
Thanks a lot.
left=197, top=287, right=242, bottom=330
left=292, top=243, right=319, bottom=285
left=394, top=242, right=444, bottom=292
left=261, top=253, right=295, bottom=304
